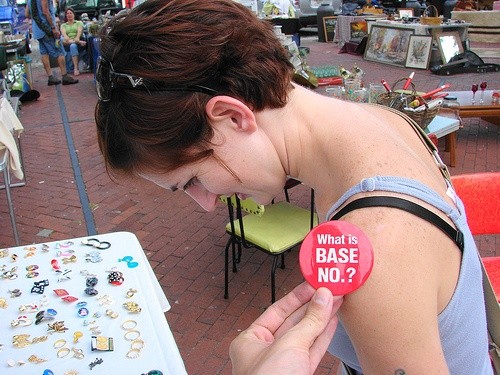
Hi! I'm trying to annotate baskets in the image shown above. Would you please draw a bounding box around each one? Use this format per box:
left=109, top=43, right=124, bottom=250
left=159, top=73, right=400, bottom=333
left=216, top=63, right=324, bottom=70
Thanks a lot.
left=418, top=5, right=443, bottom=26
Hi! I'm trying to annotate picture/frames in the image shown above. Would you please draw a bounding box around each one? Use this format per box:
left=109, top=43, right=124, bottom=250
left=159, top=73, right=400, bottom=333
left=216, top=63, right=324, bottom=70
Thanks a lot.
left=322, top=16, right=339, bottom=42
left=404, top=34, right=433, bottom=70
left=435, top=31, right=463, bottom=65
left=362, top=24, right=415, bottom=68
left=2, top=60, right=32, bottom=94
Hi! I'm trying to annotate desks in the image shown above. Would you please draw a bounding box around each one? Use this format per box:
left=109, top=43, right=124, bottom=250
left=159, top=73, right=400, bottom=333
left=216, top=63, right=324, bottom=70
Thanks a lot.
left=0, top=96, right=27, bottom=249
left=427, top=90, right=500, bottom=168
left=379, top=20, right=471, bottom=51
left=6, top=45, right=26, bottom=60
left=0, top=231, right=188, bottom=375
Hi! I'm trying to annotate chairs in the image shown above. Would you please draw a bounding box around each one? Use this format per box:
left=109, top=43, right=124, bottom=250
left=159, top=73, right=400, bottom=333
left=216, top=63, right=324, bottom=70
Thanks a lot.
left=217, top=191, right=320, bottom=305
left=450, top=171, right=500, bottom=306
left=0, top=45, right=8, bottom=92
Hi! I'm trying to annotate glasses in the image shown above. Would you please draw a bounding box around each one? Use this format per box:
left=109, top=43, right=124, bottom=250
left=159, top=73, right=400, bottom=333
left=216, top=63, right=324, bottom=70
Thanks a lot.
left=94, top=56, right=254, bottom=112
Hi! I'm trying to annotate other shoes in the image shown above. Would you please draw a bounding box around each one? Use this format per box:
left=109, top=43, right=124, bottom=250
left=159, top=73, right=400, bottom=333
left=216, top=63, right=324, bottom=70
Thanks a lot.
left=48, top=75, right=61, bottom=86
left=73, top=69, right=80, bottom=76
left=81, top=41, right=87, bottom=47
left=61, top=74, right=79, bottom=86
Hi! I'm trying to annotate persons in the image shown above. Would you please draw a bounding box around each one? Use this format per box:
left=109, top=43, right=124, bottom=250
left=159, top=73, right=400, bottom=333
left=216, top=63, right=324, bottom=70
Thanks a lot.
left=60, top=7, right=86, bottom=76
left=94, top=0, right=495, bottom=375
left=228, top=280, right=343, bottom=375
left=25, top=0, right=79, bottom=86
